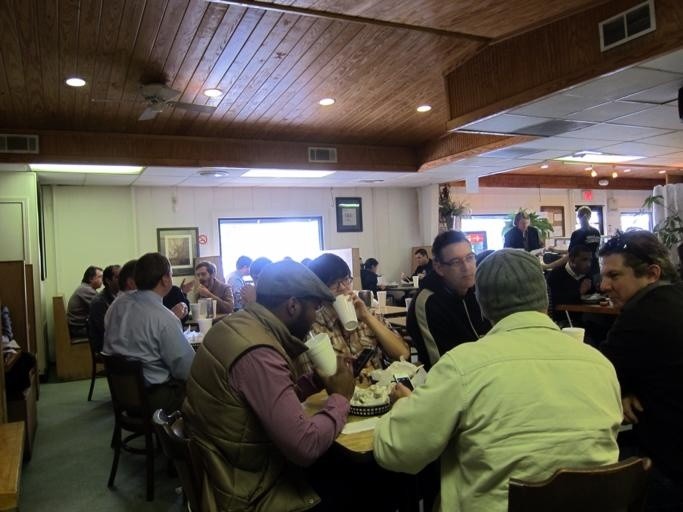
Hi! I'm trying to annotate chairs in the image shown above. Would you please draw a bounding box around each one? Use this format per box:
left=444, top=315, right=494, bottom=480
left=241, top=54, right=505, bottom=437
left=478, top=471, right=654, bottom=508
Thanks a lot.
left=100, top=350, right=180, bottom=504
left=52, top=292, right=655, bottom=401
left=152, top=409, right=199, bottom=511
left=508, top=457, right=653, bottom=508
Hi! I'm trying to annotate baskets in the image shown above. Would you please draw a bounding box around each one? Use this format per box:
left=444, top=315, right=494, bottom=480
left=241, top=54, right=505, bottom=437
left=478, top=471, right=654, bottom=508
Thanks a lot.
left=349, top=403, right=388, bottom=416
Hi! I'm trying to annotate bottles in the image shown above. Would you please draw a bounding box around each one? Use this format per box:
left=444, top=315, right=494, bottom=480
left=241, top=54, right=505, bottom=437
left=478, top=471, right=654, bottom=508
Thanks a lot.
left=234, top=291, right=240, bottom=308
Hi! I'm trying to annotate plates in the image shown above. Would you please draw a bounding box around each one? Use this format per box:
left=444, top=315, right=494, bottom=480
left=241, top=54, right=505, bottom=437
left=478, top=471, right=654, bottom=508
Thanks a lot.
left=580, top=294, right=605, bottom=303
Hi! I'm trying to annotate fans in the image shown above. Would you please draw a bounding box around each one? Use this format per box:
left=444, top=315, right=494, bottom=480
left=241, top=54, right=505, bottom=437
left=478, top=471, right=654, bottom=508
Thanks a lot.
left=89, top=82, right=216, bottom=120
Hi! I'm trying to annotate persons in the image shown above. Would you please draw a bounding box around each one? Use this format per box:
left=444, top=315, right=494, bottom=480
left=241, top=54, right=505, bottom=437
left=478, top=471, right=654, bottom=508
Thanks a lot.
left=504, top=211, right=541, bottom=253
left=548, top=207, right=600, bottom=254
left=65, top=230, right=681, bottom=512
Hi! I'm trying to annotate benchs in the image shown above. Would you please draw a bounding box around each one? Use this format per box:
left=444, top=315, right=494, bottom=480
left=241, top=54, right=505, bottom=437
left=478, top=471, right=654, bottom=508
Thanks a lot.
left=317, top=248, right=365, bottom=293
left=0, top=261, right=38, bottom=464
left=412, top=246, right=434, bottom=274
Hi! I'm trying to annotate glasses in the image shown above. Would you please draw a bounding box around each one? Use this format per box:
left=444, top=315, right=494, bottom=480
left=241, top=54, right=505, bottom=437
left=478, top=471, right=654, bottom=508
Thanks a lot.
left=608, top=237, right=652, bottom=263
left=439, top=251, right=477, bottom=266
left=326, top=276, right=353, bottom=289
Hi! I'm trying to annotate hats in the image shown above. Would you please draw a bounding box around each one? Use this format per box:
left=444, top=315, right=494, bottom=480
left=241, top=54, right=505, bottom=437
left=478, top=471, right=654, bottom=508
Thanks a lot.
left=474, top=249, right=550, bottom=321
left=256, top=259, right=336, bottom=302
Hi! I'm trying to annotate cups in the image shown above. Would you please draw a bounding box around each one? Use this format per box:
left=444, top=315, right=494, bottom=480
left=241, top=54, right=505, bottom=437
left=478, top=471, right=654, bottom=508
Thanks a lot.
left=412, top=276, right=418, bottom=286
left=332, top=293, right=358, bottom=331
left=304, top=333, right=337, bottom=376
left=377, top=290, right=386, bottom=307
left=189, top=297, right=217, bottom=336
left=562, top=326, right=585, bottom=342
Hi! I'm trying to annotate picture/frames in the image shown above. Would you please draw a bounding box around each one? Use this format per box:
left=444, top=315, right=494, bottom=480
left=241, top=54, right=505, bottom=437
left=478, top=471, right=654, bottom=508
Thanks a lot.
left=157, top=227, right=200, bottom=276
left=336, top=196, right=363, bottom=233
